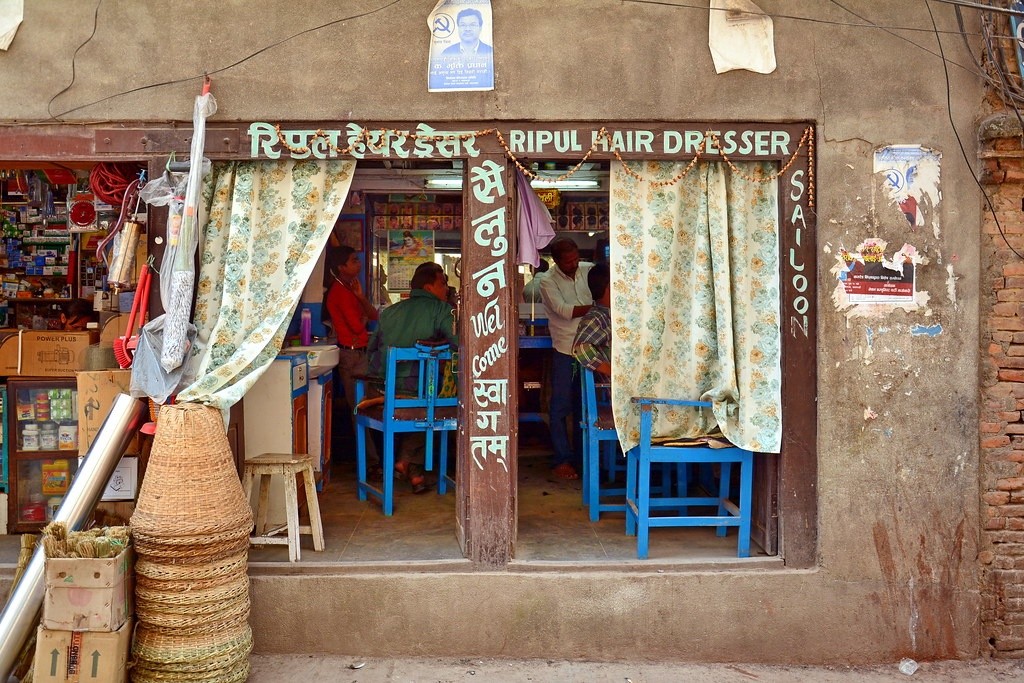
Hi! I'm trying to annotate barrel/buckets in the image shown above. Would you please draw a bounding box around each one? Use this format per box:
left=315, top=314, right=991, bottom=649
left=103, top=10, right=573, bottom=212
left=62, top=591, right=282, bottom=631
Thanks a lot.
left=41, top=459, right=70, bottom=496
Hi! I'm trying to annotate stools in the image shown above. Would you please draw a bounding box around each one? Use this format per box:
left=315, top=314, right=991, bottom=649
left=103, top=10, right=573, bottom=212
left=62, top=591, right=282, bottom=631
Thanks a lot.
left=242, top=452, right=327, bottom=561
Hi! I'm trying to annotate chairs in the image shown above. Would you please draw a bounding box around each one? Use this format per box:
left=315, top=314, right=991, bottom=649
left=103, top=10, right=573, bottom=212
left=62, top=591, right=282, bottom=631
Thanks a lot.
left=354, top=341, right=752, bottom=560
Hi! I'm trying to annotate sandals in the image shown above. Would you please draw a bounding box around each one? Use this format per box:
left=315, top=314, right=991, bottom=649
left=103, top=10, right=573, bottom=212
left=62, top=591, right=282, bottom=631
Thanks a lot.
left=409, top=471, right=437, bottom=495
left=394, top=456, right=417, bottom=481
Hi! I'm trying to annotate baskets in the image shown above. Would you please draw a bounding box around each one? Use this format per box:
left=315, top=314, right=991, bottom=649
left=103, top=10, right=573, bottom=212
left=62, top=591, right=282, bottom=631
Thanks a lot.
left=129, top=401, right=255, bottom=683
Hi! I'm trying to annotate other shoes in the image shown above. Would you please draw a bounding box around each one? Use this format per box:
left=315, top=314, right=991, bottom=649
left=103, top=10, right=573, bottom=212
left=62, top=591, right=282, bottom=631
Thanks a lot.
left=551, top=464, right=580, bottom=480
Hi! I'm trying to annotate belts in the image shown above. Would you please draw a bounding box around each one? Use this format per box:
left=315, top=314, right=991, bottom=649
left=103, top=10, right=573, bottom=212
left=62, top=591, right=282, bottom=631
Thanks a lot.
left=337, top=343, right=368, bottom=351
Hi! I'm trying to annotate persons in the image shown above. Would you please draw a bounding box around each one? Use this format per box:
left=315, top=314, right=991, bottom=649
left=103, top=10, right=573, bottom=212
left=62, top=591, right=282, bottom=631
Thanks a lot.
left=366, top=266, right=458, bottom=493
left=571, top=263, right=615, bottom=431
left=441, top=8, right=493, bottom=56
left=539, top=236, right=603, bottom=480
left=326, top=246, right=383, bottom=482
left=446, top=286, right=458, bottom=308
left=522, top=258, right=551, bottom=336
left=60, top=298, right=98, bottom=331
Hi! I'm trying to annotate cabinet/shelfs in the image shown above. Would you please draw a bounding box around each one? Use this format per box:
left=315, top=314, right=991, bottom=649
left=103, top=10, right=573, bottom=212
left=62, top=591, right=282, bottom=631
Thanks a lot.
left=7, top=375, right=78, bottom=535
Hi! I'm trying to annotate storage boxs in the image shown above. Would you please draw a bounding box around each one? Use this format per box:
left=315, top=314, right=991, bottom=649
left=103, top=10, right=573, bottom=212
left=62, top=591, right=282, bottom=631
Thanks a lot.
left=42, top=546, right=138, bottom=632
left=32, top=616, right=134, bottom=683
left=0, top=328, right=152, bottom=501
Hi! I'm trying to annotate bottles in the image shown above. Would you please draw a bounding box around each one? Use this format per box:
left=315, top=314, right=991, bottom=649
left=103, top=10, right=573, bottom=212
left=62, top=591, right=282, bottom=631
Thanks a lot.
left=41, top=184, right=55, bottom=218
left=26, top=175, right=42, bottom=207
left=300, top=308, right=311, bottom=347
